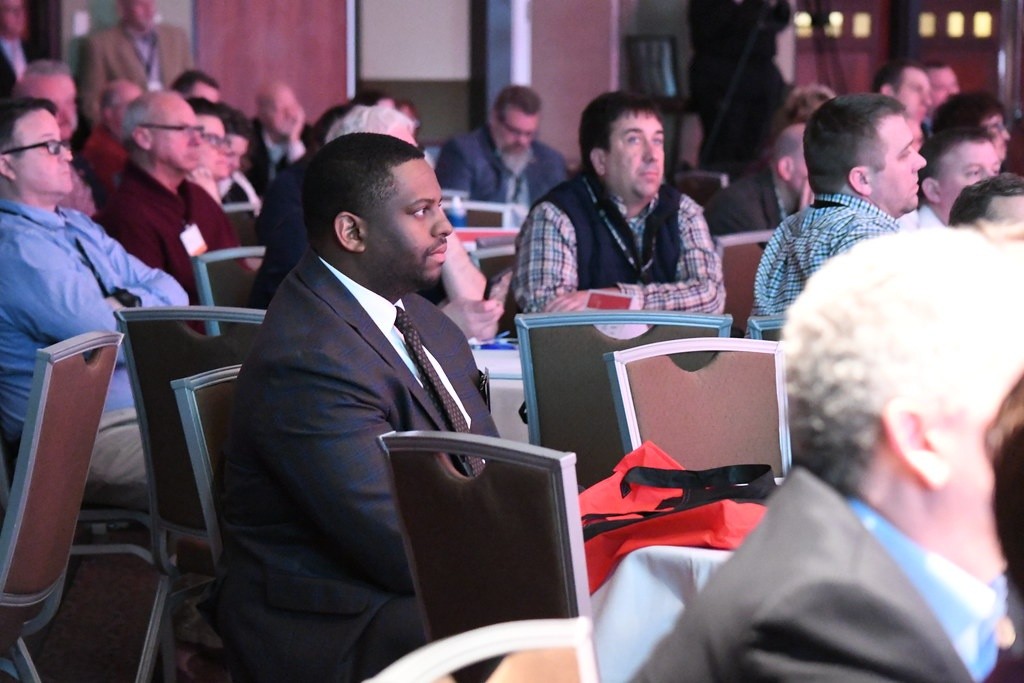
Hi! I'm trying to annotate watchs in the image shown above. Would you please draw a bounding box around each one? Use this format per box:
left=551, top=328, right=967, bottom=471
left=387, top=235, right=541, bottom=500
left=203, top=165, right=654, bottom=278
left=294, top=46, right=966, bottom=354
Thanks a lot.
left=113, top=290, right=136, bottom=307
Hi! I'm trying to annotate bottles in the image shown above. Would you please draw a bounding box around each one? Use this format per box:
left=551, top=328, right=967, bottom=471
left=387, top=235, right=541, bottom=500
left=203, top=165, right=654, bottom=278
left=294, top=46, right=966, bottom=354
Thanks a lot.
left=448, top=196, right=466, bottom=228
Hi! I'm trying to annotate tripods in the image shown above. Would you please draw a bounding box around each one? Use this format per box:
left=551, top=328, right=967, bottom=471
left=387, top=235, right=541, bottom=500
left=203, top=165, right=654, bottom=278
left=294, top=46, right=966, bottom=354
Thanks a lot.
left=698, top=0, right=849, bottom=165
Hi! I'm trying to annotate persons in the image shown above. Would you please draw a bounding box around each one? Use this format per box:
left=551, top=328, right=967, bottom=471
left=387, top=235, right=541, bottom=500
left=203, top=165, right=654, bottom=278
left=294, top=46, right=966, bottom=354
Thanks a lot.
left=0, top=59, right=1024, bottom=683
left=70, top=0, right=191, bottom=89
left=0, top=0, right=37, bottom=98
left=684, top=0, right=792, bottom=175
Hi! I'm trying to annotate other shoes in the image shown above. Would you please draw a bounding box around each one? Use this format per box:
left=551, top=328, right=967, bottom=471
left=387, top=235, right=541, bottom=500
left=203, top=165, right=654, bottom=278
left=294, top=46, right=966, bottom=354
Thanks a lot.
left=151, top=634, right=201, bottom=683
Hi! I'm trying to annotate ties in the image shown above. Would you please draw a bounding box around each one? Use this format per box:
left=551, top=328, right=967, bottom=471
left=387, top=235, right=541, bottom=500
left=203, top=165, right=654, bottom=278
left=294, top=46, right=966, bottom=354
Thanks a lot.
left=391, top=305, right=486, bottom=478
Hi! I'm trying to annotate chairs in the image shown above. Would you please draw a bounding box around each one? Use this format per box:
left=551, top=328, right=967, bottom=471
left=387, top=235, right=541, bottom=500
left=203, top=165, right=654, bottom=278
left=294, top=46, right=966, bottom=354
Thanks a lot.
left=170, top=361, right=244, bottom=683
left=748, top=314, right=785, bottom=342
left=466, top=200, right=512, bottom=229
left=602, top=338, right=793, bottom=479
left=112, top=307, right=268, bottom=683
left=469, top=244, right=523, bottom=338
left=715, top=229, right=774, bottom=338
left=0, top=330, right=125, bottom=683
left=623, top=31, right=686, bottom=186
left=191, top=244, right=268, bottom=336
left=223, top=200, right=261, bottom=246
left=377, top=427, right=593, bottom=683
left=364, top=614, right=598, bottom=683
left=514, top=311, right=734, bottom=490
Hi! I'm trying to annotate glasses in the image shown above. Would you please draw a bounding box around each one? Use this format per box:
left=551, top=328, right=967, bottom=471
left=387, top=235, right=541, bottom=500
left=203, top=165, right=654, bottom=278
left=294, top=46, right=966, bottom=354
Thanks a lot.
left=200, top=132, right=233, bottom=151
left=0, top=137, right=71, bottom=156
left=984, top=117, right=1012, bottom=136
left=137, top=118, right=205, bottom=144
left=494, top=115, right=538, bottom=143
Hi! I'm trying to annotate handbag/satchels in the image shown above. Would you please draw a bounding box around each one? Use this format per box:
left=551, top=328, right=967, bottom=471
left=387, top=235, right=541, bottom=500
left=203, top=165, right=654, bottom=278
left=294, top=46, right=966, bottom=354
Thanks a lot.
left=576, top=440, right=776, bottom=596
